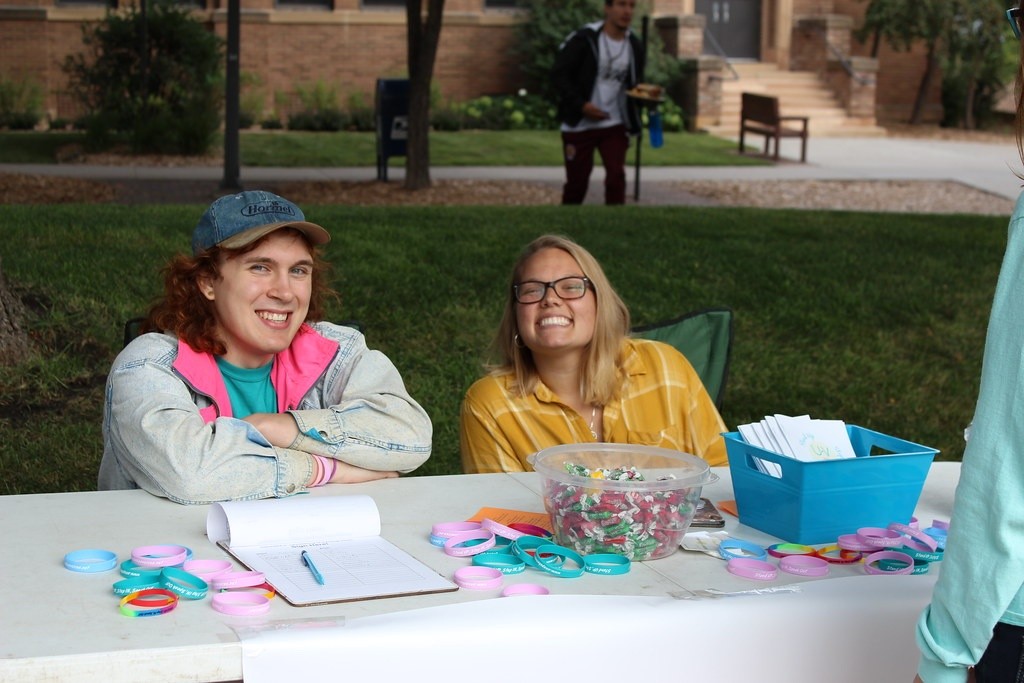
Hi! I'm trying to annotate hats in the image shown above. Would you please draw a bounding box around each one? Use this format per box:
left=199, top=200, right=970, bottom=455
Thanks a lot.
left=192, top=190, right=331, bottom=260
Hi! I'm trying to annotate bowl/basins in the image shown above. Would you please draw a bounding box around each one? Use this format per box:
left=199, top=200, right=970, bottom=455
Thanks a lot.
left=525, top=443, right=721, bottom=562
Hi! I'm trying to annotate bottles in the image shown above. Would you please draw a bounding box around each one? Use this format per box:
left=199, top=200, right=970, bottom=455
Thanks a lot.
left=649, top=105, right=664, bottom=147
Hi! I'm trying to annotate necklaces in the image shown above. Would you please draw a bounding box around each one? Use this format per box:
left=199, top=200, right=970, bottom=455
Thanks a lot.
left=588, top=406, right=599, bottom=440
left=602, top=31, right=629, bottom=80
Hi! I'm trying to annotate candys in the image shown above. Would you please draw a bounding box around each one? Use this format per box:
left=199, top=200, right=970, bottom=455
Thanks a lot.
left=541, top=460, right=700, bottom=561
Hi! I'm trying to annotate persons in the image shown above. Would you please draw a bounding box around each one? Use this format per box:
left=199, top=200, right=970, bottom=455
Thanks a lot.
left=540, top=0, right=649, bottom=206
left=461, top=235, right=734, bottom=473
left=910, top=0, right=1023, bottom=683
left=98, top=191, right=434, bottom=505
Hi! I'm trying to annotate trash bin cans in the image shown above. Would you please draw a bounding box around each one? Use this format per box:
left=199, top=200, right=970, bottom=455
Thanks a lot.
left=373, top=78, right=413, bottom=182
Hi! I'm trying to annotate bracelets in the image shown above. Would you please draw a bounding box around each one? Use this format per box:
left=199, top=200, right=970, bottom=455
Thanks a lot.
left=65, top=542, right=275, bottom=616
left=715, top=515, right=956, bottom=579
left=429, top=515, right=633, bottom=597
left=307, top=453, right=337, bottom=488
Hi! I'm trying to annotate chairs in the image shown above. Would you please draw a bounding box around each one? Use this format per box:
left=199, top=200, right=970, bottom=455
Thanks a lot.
left=739, top=92, right=809, bottom=163
left=630, top=306, right=736, bottom=411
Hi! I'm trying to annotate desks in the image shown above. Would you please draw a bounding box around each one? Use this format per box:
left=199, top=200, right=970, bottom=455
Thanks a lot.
left=0, top=458, right=963, bottom=683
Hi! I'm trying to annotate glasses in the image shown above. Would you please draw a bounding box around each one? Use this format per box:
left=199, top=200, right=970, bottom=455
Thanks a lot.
left=512, top=277, right=589, bottom=304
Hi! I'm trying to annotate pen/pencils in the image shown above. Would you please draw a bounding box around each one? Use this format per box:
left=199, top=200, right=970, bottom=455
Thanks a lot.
left=300, top=550, right=325, bottom=586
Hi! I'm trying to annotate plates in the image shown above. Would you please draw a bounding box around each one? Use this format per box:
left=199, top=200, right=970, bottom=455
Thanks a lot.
left=625, top=90, right=669, bottom=103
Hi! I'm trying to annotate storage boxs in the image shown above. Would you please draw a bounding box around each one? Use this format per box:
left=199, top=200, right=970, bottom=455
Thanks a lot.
left=718, top=423, right=941, bottom=547
